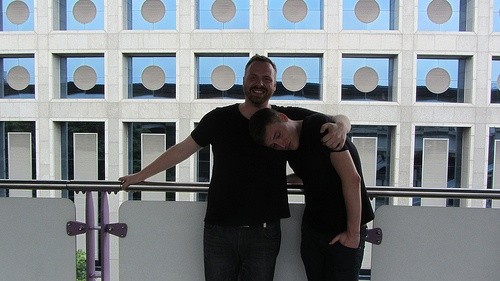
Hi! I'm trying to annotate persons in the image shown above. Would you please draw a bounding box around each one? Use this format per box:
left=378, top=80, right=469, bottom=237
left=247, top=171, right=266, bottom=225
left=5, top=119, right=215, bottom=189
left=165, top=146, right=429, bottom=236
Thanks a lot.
left=118, top=53, right=352, bottom=280
left=247, top=108, right=375, bottom=281
